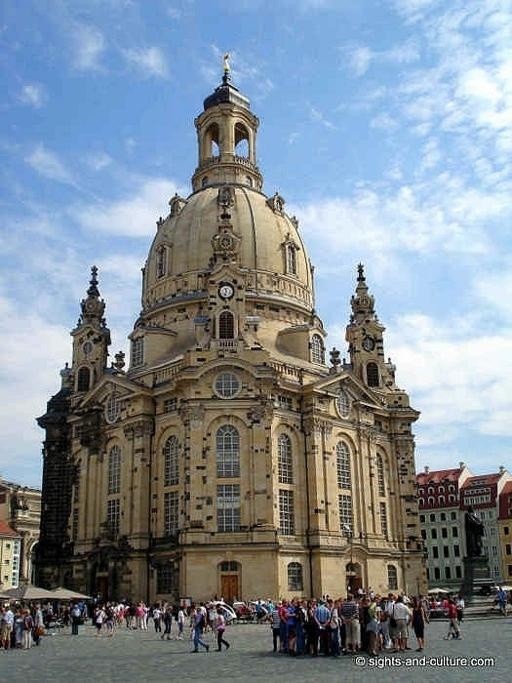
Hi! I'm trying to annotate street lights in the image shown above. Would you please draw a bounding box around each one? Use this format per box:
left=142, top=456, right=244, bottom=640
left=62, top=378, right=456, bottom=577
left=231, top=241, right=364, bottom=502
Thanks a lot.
left=343, top=523, right=354, bottom=583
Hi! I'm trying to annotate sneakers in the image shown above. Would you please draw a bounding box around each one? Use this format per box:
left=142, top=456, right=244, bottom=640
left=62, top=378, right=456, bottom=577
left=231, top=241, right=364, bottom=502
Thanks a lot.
left=271, top=642, right=426, bottom=658
left=160, top=633, right=231, bottom=653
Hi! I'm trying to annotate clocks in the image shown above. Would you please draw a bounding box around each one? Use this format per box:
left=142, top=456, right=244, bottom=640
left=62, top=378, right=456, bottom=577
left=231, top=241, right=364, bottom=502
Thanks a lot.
left=218, top=281, right=234, bottom=300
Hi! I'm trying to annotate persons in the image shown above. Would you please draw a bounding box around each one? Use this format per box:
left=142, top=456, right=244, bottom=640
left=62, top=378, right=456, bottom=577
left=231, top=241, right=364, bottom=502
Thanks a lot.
left=1, top=586, right=510, bottom=658
